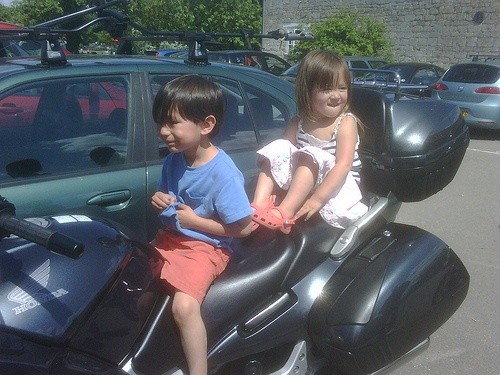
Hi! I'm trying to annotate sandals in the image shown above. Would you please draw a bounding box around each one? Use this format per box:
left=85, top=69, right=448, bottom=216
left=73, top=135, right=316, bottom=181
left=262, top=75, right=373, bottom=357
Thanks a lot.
left=252, top=207, right=296, bottom=235
left=251, top=201, right=275, bottom=232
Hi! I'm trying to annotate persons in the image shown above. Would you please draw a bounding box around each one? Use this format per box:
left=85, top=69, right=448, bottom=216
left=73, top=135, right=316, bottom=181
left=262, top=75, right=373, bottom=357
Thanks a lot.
left=148, top=73, right=253, bottom=375
left=250, top=48, right=369, bottom=234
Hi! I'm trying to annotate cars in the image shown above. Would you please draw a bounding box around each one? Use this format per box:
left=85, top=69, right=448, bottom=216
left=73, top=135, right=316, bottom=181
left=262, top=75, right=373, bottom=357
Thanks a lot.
left=431, top=61, right=500, bottom=136
left=278, top=56, right=388, bottom=84
left=178, top=50, right=292, bottom=77
left=156, top=50, right=187, bottom=58
left=0, top=22, right=162, bottom=144
left=352, top=62, right=447, bottom=99
left=0, top=54, right=304, bottom=245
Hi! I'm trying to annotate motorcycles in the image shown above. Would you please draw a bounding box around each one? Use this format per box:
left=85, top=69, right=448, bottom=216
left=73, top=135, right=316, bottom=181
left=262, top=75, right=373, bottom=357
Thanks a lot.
left=0, top=88, right=471, bottom=375
